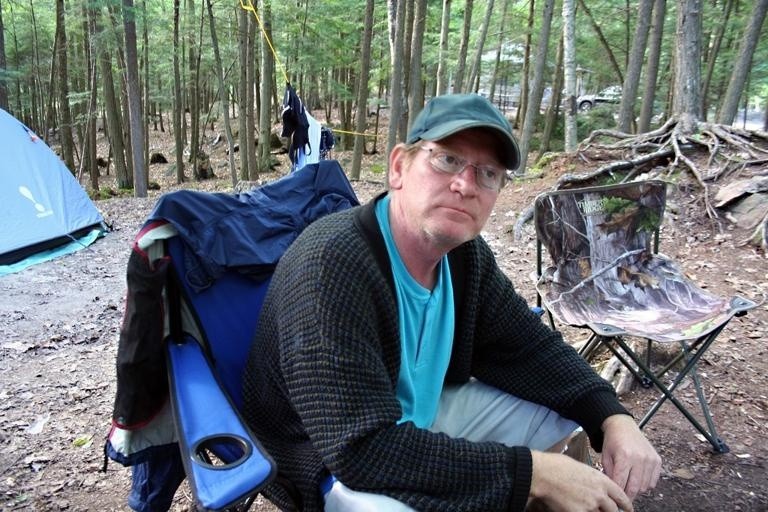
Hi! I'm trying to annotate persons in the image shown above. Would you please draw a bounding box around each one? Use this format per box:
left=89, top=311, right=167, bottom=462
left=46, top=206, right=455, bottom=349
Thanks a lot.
left=238, top=92, right=663, bottom=510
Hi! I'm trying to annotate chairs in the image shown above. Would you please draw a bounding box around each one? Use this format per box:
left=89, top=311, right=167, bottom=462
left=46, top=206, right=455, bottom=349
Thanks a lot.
left=532, top=177, right=759, bottom=455
left=158, top=159, right=360, bottom=512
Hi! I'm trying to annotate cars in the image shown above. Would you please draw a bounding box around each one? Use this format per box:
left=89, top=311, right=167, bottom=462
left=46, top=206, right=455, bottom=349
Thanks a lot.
left=576, top=85, right=623, bottom=112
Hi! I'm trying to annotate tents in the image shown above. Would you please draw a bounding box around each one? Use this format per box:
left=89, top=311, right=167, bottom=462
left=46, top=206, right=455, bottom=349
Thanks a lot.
left=1, top=108, right=109, bottom=280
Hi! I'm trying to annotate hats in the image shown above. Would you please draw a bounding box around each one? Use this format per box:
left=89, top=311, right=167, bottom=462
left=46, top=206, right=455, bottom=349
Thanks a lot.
left=405, top=92, right=522, bottom=172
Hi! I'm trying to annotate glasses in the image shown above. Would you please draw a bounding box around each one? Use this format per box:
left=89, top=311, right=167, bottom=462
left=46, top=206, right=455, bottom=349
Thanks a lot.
left=406, top=142, right=515, bottom=194
